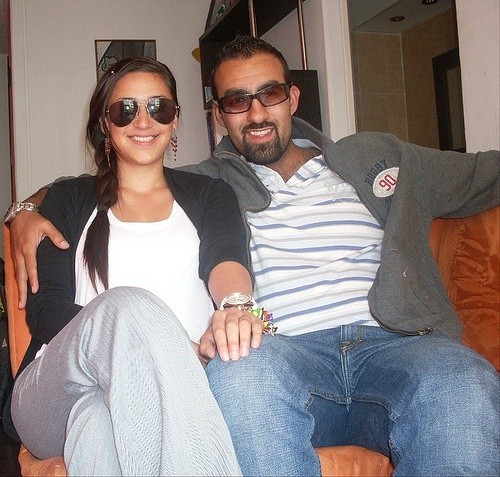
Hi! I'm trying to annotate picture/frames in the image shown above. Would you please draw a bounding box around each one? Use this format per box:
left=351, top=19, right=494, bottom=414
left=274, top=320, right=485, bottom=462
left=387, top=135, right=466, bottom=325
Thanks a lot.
left=93, top=38, right=157, bottom=82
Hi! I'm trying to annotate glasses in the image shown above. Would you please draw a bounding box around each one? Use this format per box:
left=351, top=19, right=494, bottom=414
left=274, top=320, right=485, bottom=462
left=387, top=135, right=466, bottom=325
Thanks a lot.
left=106, top=98, right=180, bottom=127
left=212, top=82, right=293, bottom=113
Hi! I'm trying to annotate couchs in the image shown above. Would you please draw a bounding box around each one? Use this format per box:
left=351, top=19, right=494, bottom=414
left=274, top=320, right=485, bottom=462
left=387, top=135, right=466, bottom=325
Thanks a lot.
left=3, top=169, right=500, bottom=477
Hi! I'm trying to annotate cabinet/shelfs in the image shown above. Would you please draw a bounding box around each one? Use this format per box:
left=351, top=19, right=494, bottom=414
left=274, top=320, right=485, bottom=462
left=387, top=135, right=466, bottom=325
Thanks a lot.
left=196, top=0, right=323, bottom=158
